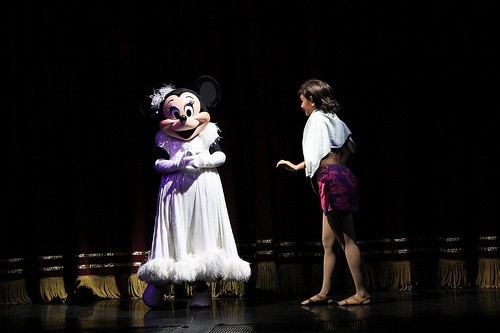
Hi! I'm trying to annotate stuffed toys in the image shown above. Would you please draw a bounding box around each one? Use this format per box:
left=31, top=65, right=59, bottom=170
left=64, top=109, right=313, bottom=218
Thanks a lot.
left=135, top=75, right=252, bottom=309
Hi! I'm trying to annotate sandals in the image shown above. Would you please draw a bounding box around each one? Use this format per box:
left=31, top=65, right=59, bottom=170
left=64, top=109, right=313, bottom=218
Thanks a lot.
left=301, top=295, right=334, bottom=306
left=338, top=295, right=372, bottom=305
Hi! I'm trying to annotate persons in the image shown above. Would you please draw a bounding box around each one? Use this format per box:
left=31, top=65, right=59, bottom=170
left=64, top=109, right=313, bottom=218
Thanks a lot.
left=276, top=79, right=377, bottom=306
